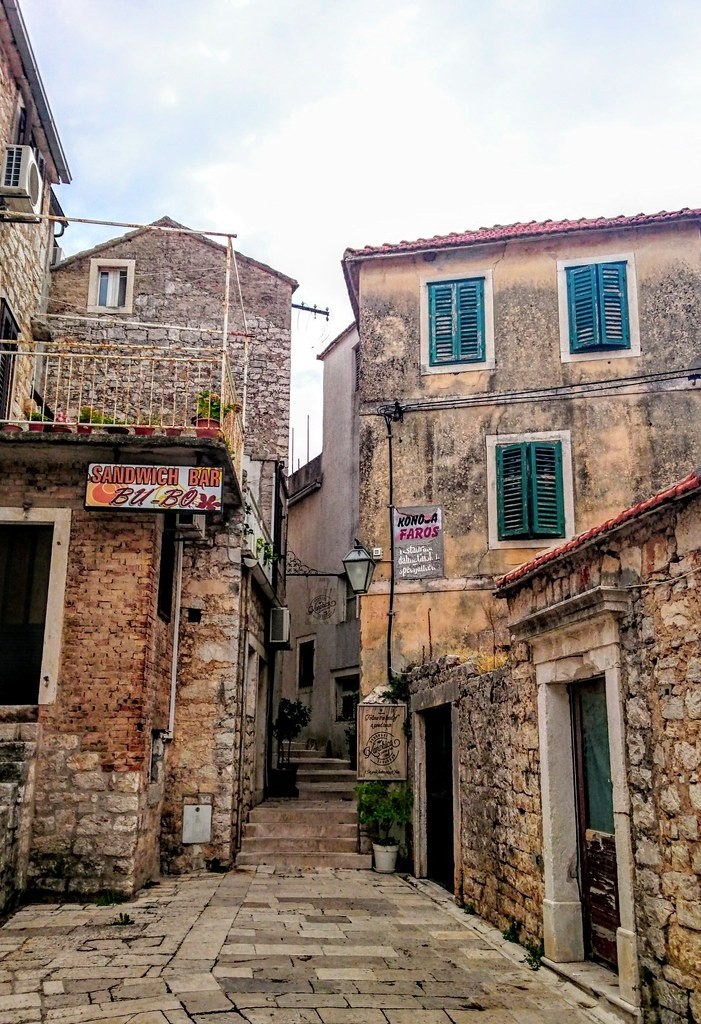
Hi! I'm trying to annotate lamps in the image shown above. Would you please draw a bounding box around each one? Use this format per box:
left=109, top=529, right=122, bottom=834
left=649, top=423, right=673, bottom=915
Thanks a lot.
left=342, top=538, right=376, bottom=594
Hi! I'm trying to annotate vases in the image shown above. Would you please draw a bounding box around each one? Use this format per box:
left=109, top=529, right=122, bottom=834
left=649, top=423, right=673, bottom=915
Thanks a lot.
left=194, top=419, right=220, bottom=438
left=53, top=426, right=72, bottom=434
left=1, top=424, right=24, bottom=433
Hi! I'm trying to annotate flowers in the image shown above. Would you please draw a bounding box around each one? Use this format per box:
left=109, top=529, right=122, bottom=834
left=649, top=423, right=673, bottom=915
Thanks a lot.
left=191, top=391, right=242, bottom=424
left=56, top=410, right=71, bottom=429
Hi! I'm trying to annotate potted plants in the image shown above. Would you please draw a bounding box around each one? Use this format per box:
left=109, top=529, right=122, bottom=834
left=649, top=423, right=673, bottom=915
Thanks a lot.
left=269, top=696, right=314, bottom=796
left=354, top=781, right=414, bottom=870
left=344, top=725, right=357, bottom=770
left=28, top=413, right=54, bottom=432
left=74, top=405, right=185, bottom=436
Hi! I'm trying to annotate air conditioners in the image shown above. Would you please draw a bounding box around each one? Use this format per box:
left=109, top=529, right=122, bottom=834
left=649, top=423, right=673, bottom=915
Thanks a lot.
left=270, top=606, right=290, bottom=643
left=0, top=143, right=44, bottom=222
left=176, top=513, right=205, bottom=538
